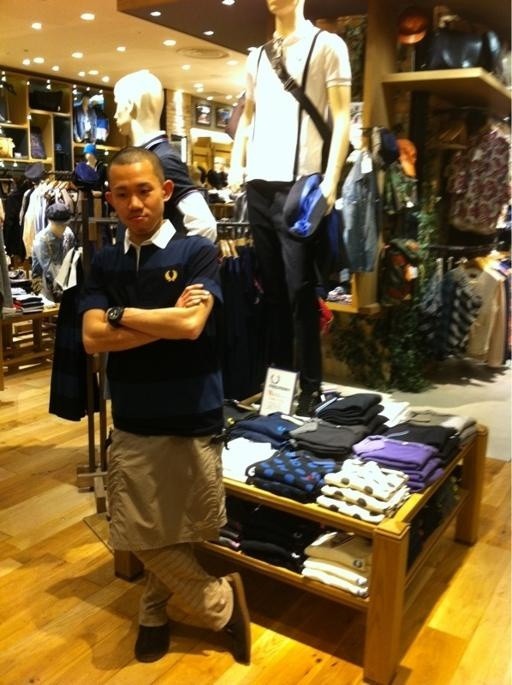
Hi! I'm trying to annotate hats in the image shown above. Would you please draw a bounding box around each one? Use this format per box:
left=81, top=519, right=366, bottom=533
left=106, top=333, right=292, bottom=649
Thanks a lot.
left=370, top=125, right=399, bottom=170
left=82, top=143, right=98, bottom=157
left=46, top=202, right=71, bottom=222
left=394, top=136, right=419, bottom=178
left=395, top=11, right=430, bottom=46
left=24, top=163, right=45, bottom=181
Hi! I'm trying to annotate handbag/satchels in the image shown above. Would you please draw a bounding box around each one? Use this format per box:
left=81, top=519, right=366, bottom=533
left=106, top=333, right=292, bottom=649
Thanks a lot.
left=317, top=131, right=360, bottom=201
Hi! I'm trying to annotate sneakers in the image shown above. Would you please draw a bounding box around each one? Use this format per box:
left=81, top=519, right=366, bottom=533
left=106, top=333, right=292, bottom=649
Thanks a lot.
left=133, top=620, right=172, bottom=664
left=217, top=571, right=254, bottom=668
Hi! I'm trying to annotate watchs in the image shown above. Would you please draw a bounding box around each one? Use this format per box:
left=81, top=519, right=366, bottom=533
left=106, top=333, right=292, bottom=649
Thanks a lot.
left=107, top=305, right=124, bottom=327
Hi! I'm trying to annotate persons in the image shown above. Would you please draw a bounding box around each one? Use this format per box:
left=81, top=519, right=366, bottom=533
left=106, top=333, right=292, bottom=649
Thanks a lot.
left=74, top=146, right=253, bottom=664
left=93, top=104, right=111, bottom=144
left=29, top=204, right=78, bottom=295
left=114, top=69, right=218, bottom=241
left=73, top=91, right=97, bottom=143
left=229, top=0, right=353, bottom=414
left=83, top=144, right=105, bottom=176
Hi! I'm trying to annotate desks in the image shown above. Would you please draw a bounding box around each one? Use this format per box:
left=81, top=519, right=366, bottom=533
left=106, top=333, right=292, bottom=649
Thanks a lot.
left=179, top=380, right=489, bottom=685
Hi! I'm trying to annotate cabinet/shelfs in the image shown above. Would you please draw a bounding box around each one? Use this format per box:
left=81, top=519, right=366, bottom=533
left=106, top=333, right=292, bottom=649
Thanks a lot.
left=3, top=69, right=127, bottom=369
left=386, top=69, right=506, bottom=364
left=166, top=89, right=241, bottom=219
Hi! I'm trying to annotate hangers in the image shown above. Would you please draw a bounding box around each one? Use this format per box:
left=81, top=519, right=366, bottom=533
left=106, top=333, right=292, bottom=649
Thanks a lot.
left=215, top=221, right=254, bottom=258
